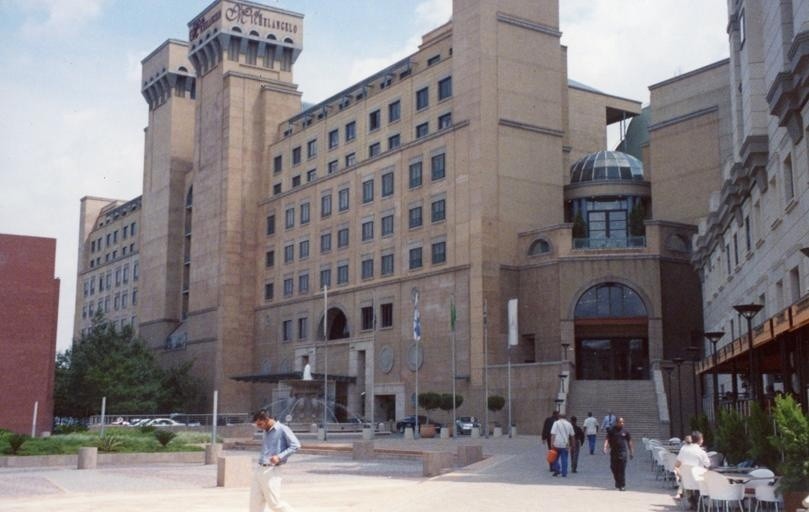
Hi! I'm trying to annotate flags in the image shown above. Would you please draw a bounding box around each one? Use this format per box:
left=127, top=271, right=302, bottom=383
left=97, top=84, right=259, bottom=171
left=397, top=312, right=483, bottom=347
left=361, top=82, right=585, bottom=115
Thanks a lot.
left=413, top=291, right=421, bottom=342
left=483, top=299, right=488, bottom=330
left=450, top=294, right=457, bottom=335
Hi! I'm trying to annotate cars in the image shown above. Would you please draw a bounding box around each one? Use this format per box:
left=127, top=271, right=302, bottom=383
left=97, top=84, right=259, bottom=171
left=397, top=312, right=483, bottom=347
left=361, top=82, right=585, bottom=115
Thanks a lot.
left=397, top=415, right=446, bottom=433
left=112, top=418, right=201, bottom=427
left=456, top=416, right=481, bottom=435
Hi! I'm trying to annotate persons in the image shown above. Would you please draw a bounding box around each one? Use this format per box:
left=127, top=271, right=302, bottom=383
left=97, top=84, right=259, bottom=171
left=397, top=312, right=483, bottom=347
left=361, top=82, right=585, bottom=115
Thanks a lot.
left=540, top=410, right=635, bottom=491
left=675, top=430, right=710, bottom=511
left=249, top=407, right=302, bottom=512
left=674, top=436, right=693, bottom=499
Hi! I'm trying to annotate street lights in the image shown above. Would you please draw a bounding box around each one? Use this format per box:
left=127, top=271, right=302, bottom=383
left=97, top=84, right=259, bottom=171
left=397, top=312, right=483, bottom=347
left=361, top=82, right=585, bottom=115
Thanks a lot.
left=733, top=305, right=763, bottom=400
left=704, top=332, right=725, bottom=428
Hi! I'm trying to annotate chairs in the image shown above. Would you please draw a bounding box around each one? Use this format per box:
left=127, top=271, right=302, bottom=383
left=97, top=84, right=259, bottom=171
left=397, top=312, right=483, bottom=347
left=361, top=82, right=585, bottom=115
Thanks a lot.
left=639, top=436, right=784, bottom=512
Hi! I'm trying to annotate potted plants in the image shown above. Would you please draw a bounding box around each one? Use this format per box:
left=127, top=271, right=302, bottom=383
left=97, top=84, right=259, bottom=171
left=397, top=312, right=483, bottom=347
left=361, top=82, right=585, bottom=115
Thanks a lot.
left=691, top=393, right=808, bottom=512
left=417, top=392, right=440, bottom=438
left=440, top=393, right=463, bottom=437
left=489, top=396, right=505, bottom=434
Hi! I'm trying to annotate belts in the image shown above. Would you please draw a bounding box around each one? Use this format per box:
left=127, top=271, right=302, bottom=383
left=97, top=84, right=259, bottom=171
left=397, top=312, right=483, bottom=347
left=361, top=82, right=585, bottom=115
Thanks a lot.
left=259, top=463, right=276, bottom=466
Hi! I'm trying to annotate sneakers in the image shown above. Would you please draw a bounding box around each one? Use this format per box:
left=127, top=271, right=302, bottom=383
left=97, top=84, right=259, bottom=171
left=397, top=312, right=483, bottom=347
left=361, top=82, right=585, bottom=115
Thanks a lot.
left=615, top=485, right=626, bottom=491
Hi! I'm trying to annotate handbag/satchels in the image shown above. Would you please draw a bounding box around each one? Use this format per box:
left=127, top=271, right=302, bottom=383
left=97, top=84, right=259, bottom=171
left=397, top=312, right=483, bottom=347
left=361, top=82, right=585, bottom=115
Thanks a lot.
left=547, top=449, right=558, bottom=464
